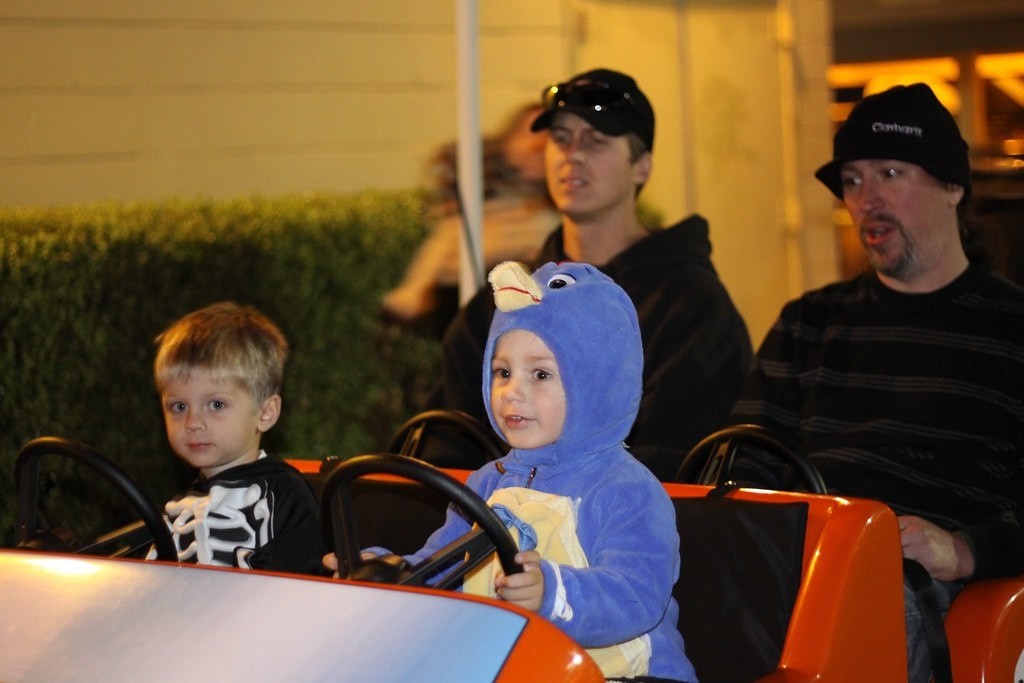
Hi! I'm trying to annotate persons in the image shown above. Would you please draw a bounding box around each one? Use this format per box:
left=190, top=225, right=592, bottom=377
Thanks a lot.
left=140, top=301, right=332, bottom=578
left=707, top=83, right=1024, bottom=683
left=323, top=260, right=699, bottom=683
left=375, top=65, right=753, bottom=482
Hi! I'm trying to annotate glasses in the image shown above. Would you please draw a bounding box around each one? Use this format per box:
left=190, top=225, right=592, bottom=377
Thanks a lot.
left=542, top=83, right=623, bottom=119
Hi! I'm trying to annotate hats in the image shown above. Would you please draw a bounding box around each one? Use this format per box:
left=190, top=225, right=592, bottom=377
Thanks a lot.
left=814, top=83, right=971, bottom=211
left=529, top=68, right=655, bottom=153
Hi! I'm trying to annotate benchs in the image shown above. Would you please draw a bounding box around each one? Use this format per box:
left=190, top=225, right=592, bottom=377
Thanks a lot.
left=290, top=455, right=902, bottom=683
